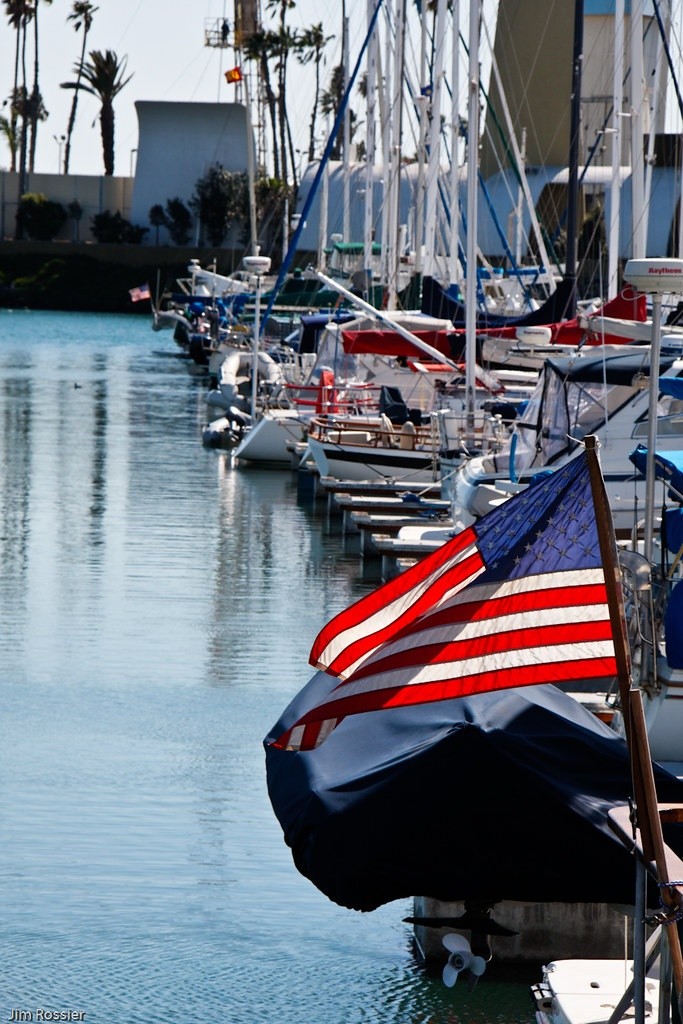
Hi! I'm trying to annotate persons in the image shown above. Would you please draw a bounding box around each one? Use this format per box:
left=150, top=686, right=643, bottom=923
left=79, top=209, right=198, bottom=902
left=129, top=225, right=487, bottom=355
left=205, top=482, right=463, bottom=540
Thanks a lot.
left=220, top=20, right=230, bottom=47
left=400, top=408, right=422, bottom=450
left=664, top=301, right=683, bottom=327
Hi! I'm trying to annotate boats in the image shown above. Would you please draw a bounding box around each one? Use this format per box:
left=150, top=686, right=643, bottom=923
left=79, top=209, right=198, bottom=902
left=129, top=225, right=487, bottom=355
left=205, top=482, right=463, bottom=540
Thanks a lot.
left=152, top=1, right=683, bottom=1024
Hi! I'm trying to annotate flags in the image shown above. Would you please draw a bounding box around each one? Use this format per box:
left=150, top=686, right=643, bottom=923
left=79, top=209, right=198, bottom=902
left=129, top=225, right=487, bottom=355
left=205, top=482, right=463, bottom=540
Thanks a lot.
left=259, top=450, right=632, bottom=752
left=225, top=67, right=242, bottom=83
left=129, top=285, right=150, bottom=303
